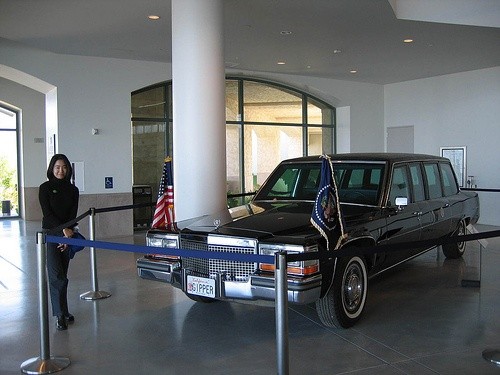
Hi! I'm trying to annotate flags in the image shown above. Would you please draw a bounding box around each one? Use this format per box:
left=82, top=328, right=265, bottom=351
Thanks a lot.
left=151, top=155, right=176, bottom=229
left=310, top=155, right=344, bottom=261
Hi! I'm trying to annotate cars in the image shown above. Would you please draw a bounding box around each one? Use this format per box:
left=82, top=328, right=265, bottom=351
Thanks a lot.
left=136, top=153, right=480, bottom=329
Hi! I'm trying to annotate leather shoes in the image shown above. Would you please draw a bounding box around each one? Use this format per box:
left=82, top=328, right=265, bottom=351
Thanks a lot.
left=65, top=312, right=74, bottom=321
left=56, top=315, right=67, bottom=330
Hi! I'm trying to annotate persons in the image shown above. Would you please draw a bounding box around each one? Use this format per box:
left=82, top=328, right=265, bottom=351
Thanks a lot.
left=38, top=154, right=80, bottom=331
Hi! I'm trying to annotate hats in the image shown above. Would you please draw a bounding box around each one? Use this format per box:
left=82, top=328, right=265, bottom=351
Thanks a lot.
left=68, top=232, right=86, bottom=260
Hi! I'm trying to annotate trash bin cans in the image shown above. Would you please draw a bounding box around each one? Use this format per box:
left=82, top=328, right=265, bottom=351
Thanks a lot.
left=133, top=185, right=153, bottom=228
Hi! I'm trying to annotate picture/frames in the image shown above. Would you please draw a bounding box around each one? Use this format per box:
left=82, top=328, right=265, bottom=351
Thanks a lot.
left=440, top=147, right=467, bottom=188
